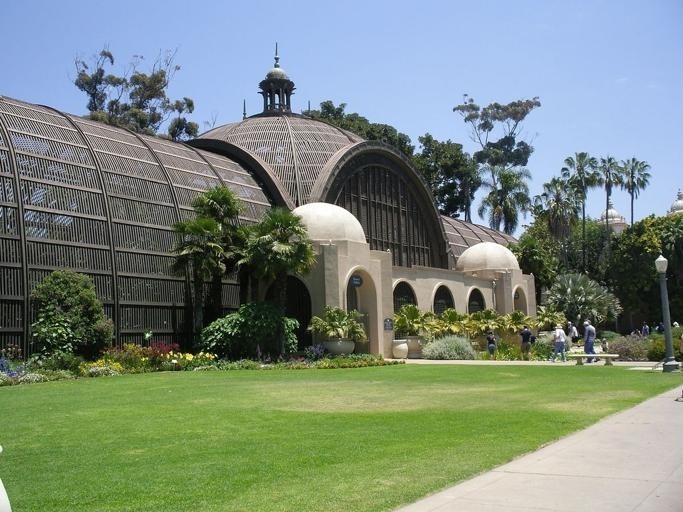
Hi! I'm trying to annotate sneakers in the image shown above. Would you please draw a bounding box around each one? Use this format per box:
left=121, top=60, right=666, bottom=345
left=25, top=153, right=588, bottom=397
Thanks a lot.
left=586, top=358, right=600, bottom=364
left=550, top=359, right=566, bottom=363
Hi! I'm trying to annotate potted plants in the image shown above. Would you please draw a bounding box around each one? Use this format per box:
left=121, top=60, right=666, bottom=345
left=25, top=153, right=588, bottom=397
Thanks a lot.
left=393, top=305, right=429, bottom=359
left=306, top=304, right=369, bottom=354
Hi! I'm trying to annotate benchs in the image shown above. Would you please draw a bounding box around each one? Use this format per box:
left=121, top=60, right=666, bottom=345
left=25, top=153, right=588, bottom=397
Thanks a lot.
left=568, top=354, right=619, bottom=365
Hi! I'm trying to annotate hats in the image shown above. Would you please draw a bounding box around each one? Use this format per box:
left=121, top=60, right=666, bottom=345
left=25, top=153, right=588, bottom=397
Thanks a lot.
left=554, top=323, right=563, bottom=329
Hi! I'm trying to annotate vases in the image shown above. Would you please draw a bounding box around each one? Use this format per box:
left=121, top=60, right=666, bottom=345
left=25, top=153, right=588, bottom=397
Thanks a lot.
left=392, top=339, right=408, bottom=358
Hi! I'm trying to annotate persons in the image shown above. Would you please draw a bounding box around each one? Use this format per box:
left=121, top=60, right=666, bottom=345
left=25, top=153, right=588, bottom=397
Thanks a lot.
left=548, top=323, right=567, bottom=362
left=567, top=323, right=578, bottom=343
left=582, top=321, right=594, bottom=363
left=600, top=338, right=608, bottom=352
left=485, top=330, right=496, bottom=360
left=585, top=319, right=600, bottom=362
left=518, top=325, right=532, bottom=360
left=566, top=321, right=572, bottom=335
left=630, top=320, right=679, bottom=337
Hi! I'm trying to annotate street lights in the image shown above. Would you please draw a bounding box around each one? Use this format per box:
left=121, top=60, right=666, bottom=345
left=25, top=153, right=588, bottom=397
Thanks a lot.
left=654, top=253, right=678, bottom=373
